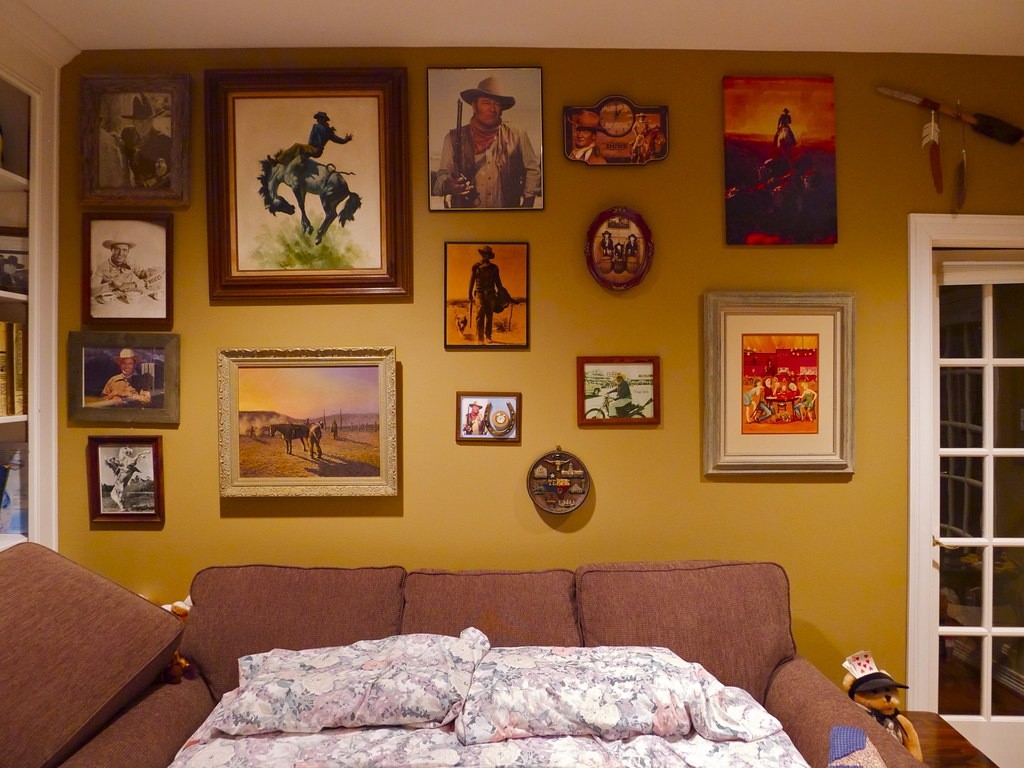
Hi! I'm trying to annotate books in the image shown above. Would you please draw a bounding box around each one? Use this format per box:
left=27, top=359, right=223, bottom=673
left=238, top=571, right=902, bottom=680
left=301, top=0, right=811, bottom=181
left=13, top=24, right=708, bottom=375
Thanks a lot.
left=0, top=442, right=28, bottom=534
left=0, top=321, right=27, bottom=416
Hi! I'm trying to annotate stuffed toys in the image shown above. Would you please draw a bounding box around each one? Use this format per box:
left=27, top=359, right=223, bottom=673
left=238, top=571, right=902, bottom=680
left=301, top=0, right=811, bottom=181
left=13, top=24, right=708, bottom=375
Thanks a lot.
left=843, top=669, right=922, bottom=761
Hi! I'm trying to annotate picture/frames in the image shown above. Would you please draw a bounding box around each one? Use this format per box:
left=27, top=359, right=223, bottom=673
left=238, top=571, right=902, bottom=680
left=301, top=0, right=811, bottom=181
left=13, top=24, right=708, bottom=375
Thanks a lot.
left=81, top=212, right=174, bottom=327
left=704, top=289, right=854, bottom=474
left=217, top=347, right=398, bottom=497
left=577, top=356, right=662, bottom=425
left=427, top=66, right=544, bottom=211
left=87, top=435, right=165, bottom=525
left=584, top=206, right=655, bottom=292
left=456, top=390, right=522, bottom=443
left=203, top=66, right=413, bottom=299
left=67, top=331, right=181, bottom=426
left=78, top=75, right=189, bottom=207
left=443, top=241, right=530, bottom=350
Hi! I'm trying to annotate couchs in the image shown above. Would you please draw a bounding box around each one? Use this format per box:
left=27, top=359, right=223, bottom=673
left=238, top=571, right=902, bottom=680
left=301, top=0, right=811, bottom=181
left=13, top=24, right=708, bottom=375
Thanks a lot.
left=58, top=558, right=931, bottom=768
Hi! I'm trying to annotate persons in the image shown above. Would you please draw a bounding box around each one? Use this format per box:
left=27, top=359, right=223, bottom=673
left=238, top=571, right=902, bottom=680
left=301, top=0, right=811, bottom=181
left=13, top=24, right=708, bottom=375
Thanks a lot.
left=105, top=457, right=124, bottom=475
left=102, top=349, right=150, bottom=406
left=0, top=255, right=25, bottom=292
left=469, top=245, right=503, bottom=343
left=91, top=230, right=147, bottom=296
left=607, top=371, right=632, bottom=418
left=130, top=475, right=150, bottom=484
left=99, top=92, right=171, bottom=190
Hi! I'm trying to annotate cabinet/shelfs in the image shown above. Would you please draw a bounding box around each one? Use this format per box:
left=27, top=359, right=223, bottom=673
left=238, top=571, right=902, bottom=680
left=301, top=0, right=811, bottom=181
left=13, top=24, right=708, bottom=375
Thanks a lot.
left=0, top=64, right=39, bottom=554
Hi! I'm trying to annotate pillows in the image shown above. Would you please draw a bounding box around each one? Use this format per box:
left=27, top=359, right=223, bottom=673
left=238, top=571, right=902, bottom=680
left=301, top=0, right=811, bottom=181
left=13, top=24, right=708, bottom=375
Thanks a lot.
left=213, top=627, right=782, bottom=743
left=0, top=541, right=185, bottom=768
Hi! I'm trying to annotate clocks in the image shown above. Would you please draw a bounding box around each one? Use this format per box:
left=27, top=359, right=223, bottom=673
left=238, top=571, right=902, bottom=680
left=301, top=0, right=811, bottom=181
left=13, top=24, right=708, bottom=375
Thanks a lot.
left=600, top=100, right=637, bottom=136
